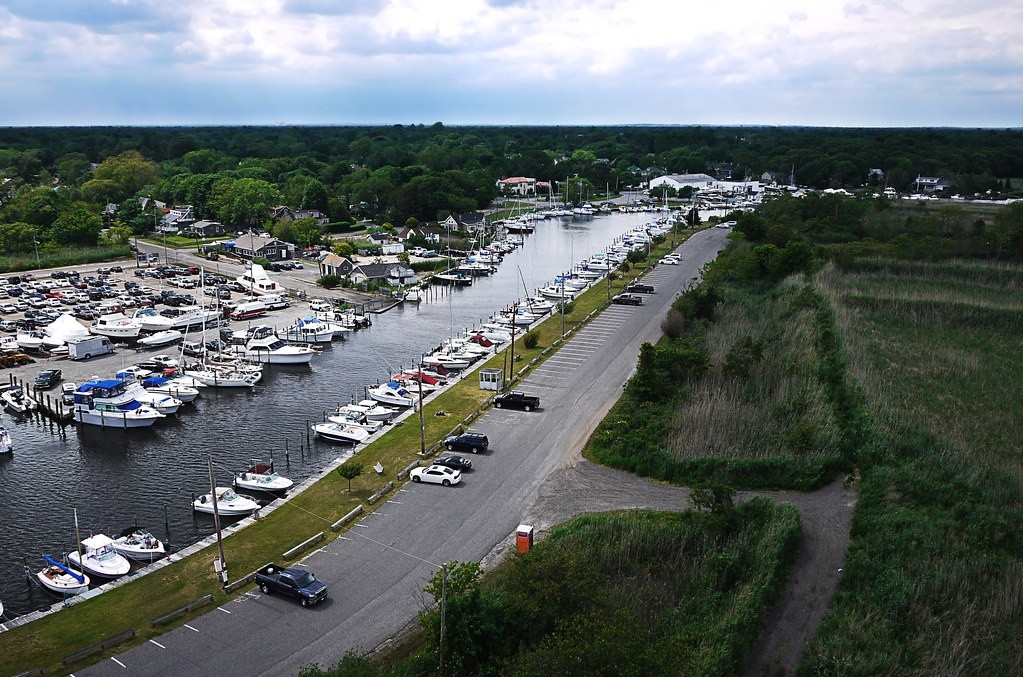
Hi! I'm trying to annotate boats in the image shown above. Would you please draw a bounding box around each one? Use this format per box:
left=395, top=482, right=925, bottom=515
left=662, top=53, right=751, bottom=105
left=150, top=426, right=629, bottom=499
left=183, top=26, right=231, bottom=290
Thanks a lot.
left=37, top=527, right=166, bottom=596
left=238, top=265, right=289, bottom=294
left=686, top=183, right=964, bottom=210
left=1, top=312, right=370, bottom=452
left=192, top=462, right=294, bottom=517
left=317, top=326, right=514, bottom=442
left=494, top=257, right=614, bottom=326
left=433, top=200, right=691, bottom=287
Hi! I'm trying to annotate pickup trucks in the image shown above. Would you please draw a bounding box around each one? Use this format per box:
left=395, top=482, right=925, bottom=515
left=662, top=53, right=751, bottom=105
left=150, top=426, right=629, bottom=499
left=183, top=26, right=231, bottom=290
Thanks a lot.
left=612, top=293, right=642, bottom=306
left=627, top=283, right=654, bottom=294
left=493, top=393, right=540, bottom=412
left=253, top=564, right=328, bottom=608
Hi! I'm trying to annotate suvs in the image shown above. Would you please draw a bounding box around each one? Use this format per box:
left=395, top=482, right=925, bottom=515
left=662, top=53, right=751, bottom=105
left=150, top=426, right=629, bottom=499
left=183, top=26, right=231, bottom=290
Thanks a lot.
left=309, top=298, right=330, bottom=314
left=444, top=433, right=488, bottom=454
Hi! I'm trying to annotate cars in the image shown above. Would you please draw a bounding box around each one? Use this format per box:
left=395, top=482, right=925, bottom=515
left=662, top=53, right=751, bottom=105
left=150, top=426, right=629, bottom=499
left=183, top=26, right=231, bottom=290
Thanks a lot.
left=659, top=253, right=682, bottom=264
left=715, top=221, right=738, bottom=229
left=0, top=265, right=287, bottom=388
left=409, top=246, right=436, bottom=259
left=290, top=261, right=303, bottom=269
left=410, top=456, right=471, bottom=487
